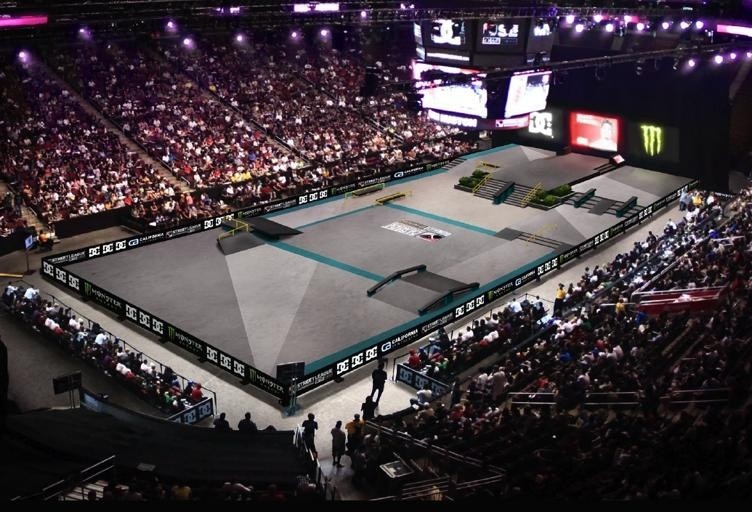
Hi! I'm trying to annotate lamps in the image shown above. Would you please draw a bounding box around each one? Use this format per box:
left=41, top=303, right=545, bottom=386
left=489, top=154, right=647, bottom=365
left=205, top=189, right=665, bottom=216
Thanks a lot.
left=535, top=4, right=752, bottom=78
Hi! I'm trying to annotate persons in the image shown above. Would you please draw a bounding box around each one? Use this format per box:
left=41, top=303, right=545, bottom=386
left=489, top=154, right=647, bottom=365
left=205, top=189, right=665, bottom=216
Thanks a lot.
left=0, top=32, right=752, bottom=512
